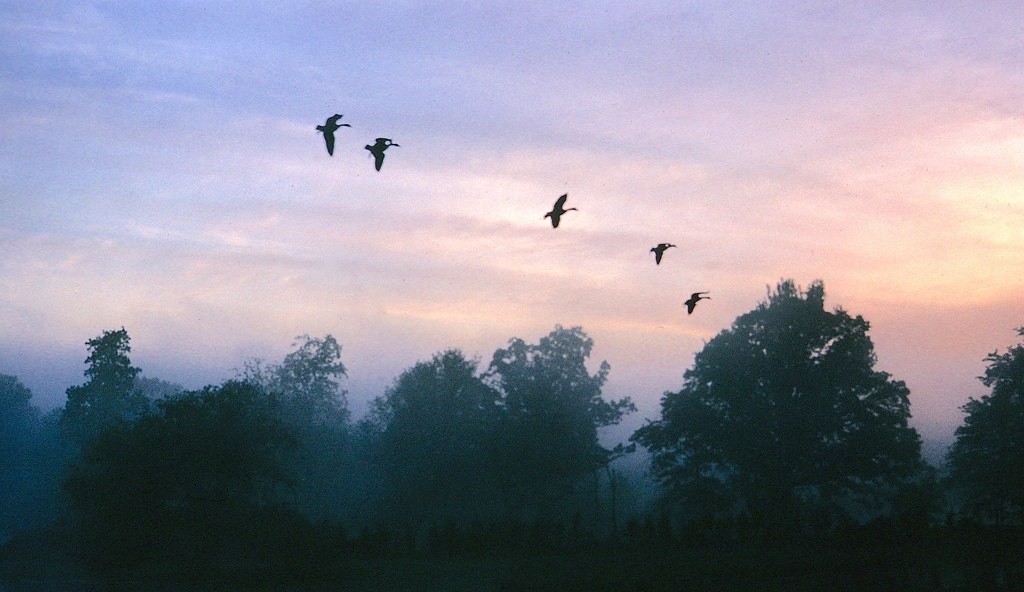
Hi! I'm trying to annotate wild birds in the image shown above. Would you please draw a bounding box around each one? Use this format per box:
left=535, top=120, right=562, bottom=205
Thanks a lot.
left=651, top=242, right=677, bottom=266
left=684, top=291, right=711, bottom=315
left=365, top=137, right=400, bottom=172
left=543, top=194, right=577, bottom=228
left=644, top=416, right=663, bottom=428
left=316, top=113, right=352, bottom=156
